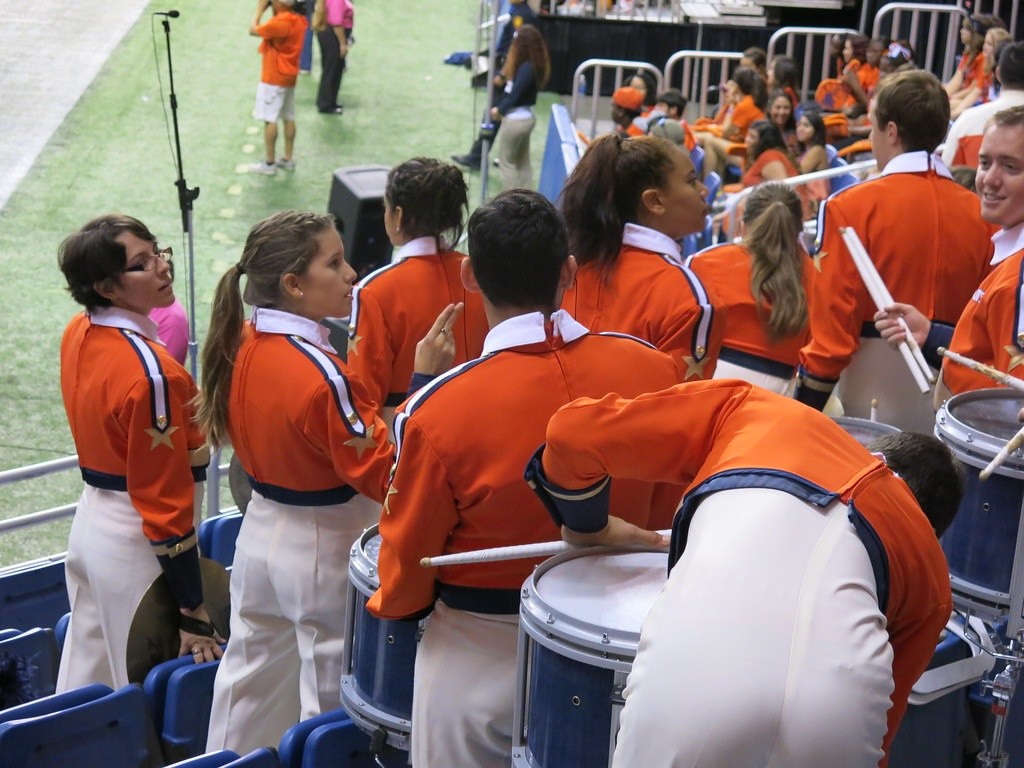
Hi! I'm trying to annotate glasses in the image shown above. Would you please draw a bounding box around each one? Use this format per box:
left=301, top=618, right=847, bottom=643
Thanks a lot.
left=116, top=247, right=174, bottom=274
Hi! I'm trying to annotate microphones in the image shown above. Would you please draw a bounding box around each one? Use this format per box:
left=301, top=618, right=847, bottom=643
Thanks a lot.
left=155, top=10, right=180, bottom=18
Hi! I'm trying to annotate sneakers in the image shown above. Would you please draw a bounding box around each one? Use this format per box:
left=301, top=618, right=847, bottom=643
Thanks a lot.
left=253, top=162, right=276, bottom=176
left=277, top=158, right=295, bottom=171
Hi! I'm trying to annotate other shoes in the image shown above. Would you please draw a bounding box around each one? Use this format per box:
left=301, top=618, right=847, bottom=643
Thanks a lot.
left=452, top=154, right=480, bottom=171
left=321, top=102, right=342, bottom=114
left=297, top=69, right=311, bottom=75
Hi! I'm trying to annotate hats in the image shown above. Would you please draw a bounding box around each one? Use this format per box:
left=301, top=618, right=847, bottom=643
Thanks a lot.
left=612, top=86, right=643, bottom=113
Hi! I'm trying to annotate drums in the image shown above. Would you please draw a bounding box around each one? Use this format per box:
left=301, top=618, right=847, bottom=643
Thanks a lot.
left=511, top=539, right=667, bottom=768
left=337, top=524, right=431, bottom=751
left=928, top=386, right=1024, bottom=623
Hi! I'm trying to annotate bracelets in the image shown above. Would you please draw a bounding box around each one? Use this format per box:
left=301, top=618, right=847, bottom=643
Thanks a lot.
left=498, top=72, right=506, bottom=81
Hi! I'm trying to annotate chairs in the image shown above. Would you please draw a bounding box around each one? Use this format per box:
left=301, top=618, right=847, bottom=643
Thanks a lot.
left=683, top=103, right=857, bottom=257
left=0, top=510, right=370, bottom=768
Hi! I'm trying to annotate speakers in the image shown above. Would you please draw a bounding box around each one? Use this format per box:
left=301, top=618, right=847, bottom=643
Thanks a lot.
left=327, top=165, right=395, bottom=284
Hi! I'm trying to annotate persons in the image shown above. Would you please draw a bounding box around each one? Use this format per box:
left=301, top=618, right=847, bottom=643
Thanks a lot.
left=189, top=211, right=465, bottom=762
left=249, top=0, right=354, bottom=175
left=53, top=214, right=227, bottom=697
left=451, top=0, right=534, bottom=170
left=147, top=260, right=187, bottom=366
left=558, top=132, right=728, bottom=385
left=523, top=380, right=963, bottom=768
left=366, top=189, right=681, bottom=768
left=490, top=26, right=550, bottom=191
left=611, top=13, right=1024, bottom=437
left=344, top=158, right=491, bottom=535
left=872, top=106, right=1024, bottom=593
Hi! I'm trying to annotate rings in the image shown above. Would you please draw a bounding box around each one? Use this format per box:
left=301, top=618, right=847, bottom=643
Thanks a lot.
left=193, top=649, right=202, bottom=655
left=441, top=329, right=448, bottom=334
